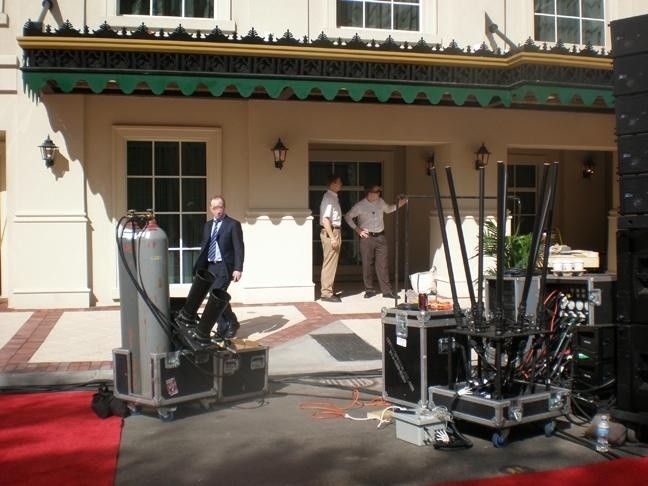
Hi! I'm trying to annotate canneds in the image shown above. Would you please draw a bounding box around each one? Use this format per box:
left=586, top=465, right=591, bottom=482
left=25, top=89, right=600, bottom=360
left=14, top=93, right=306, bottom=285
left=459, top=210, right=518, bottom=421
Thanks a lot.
left=418, top=294, right=428, bottom=311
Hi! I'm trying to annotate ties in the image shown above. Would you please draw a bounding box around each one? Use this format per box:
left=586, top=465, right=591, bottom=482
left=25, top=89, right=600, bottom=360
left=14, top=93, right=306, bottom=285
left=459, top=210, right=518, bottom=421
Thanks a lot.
left=208, top=220, right=219, bottom=261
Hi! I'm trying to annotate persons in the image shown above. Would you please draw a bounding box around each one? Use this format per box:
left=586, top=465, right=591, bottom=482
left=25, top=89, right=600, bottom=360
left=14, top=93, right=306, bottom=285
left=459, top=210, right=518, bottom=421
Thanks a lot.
left=190, top=194, right=245, bottom=344
left=318, top=170, right=343, bottom=304
left=343, top=181, right=409, bottom=301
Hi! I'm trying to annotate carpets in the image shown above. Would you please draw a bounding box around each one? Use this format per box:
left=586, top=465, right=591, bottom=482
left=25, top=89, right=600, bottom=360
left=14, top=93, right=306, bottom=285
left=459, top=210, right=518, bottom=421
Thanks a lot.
left=429, top=456, right=648, bottom=485
left=0, top=390, right=124, bottom=486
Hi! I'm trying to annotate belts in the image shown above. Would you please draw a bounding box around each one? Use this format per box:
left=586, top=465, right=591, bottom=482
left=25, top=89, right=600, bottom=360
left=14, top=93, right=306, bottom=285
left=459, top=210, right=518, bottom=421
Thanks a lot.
left=206, top=261, right=221, bottom=264
left=367, top=230, right=384, bottom=237
left=319, top=225, right=342, bottom=230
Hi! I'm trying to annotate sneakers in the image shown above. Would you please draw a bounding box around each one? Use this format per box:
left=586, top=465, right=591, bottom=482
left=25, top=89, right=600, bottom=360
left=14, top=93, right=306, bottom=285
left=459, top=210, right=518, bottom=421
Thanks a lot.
left=364, top=291, right=376, bottom=298
left=320, top=293, right=342, bottom=302
left=211, top=330, right=223, bottom=341
left=382, top=292, right=401, bottom=299
left=225, top=321, right=240, bottom=338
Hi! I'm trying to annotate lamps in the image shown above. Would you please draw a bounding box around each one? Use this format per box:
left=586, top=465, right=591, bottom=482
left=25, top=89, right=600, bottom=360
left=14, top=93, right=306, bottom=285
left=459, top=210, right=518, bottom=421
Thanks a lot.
left=37, top=134, right=60, bottom=169
left=582, top=158, right=595, bottom=178
left=475, top=143, right=491, bottom=170
left=270, top=137, right=289, bottom=170
left=426, top=152, right=434, bottom=176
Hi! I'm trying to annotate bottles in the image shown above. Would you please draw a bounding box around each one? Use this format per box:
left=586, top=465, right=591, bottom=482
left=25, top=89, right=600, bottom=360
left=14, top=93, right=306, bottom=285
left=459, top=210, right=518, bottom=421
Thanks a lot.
left=595, top=415, right=610, bottom=453
